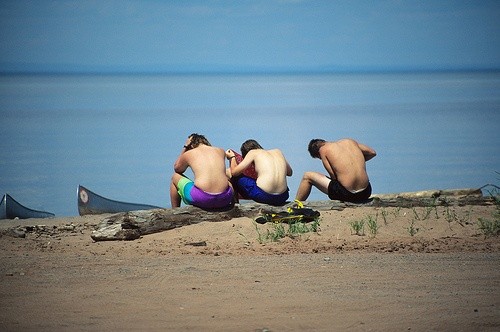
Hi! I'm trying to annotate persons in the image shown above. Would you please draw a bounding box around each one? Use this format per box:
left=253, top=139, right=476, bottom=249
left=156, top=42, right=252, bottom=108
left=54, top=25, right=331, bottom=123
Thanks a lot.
left=171, top=133, right=234, bottom=208
left=295, top=138, right=376, bottom=204
left=226, top=139, right=293, bottom=206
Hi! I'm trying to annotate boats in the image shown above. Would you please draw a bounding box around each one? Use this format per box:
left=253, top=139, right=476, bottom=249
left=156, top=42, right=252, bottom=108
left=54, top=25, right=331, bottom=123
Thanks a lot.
left=0, top=192, right=57, bottom=219
left=76, top=184, right=168, bottom=216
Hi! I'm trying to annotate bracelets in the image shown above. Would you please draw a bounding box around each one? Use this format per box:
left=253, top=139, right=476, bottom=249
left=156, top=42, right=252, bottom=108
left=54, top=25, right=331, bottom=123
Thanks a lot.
left=228, top=156, right=236, bottom=160
left=184, top=145, right=188, bottom=148
